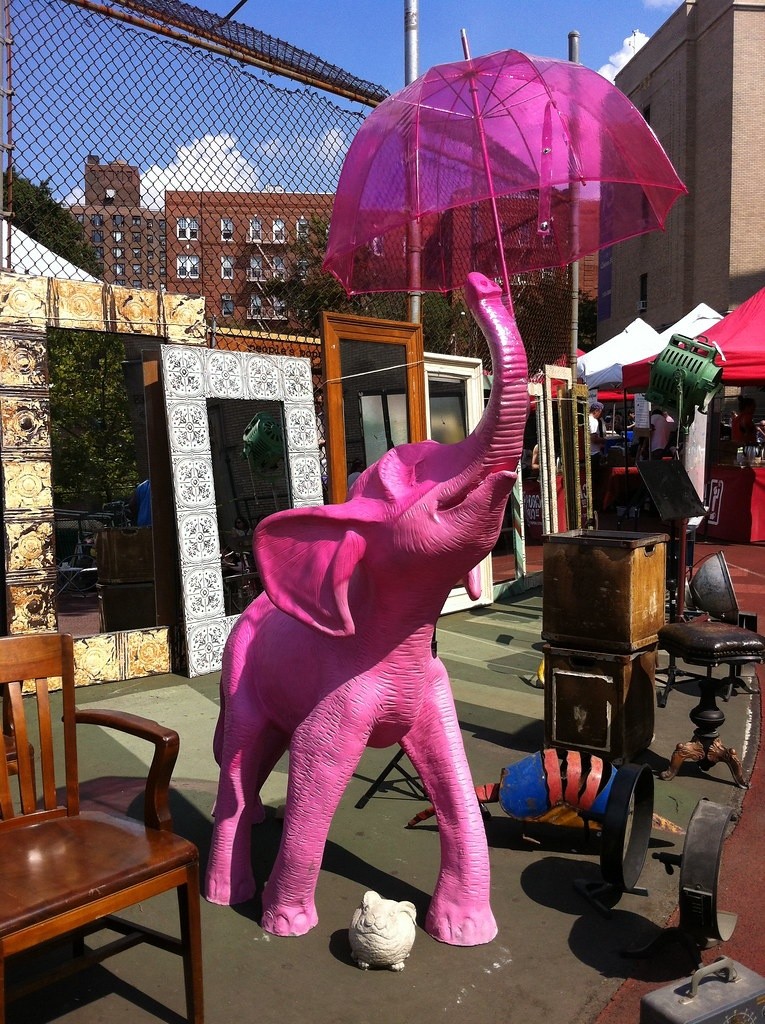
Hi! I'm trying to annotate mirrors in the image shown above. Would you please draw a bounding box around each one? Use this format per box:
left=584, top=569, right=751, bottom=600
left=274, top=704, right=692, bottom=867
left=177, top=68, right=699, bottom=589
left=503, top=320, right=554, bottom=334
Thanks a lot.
left=320, top=310, right=428, bottom=505
left=160, top=345, right=326, bottom=678
left=0, top=273, right=205, bottom=695
left=423, top=350, right=494, bottom=616
left=483, top=365, right=592, bottom=605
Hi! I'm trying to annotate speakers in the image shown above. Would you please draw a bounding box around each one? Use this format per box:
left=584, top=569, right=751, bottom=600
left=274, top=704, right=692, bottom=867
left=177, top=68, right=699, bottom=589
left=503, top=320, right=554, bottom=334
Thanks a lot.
left=95, top=527, right=155, bottom=585
left=96, top=584, right=157, bottom=635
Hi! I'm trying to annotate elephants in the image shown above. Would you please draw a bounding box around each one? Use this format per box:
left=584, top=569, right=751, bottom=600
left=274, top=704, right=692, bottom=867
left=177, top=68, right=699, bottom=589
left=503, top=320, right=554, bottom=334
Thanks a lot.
left=203, top=273, right=529, bottom=941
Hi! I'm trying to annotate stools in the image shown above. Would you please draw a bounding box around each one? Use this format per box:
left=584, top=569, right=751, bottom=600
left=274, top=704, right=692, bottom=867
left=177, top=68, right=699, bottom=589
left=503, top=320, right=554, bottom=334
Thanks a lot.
left=55, top=568, right=86, bottom=598
left=655, top=621, right=765, bottom=791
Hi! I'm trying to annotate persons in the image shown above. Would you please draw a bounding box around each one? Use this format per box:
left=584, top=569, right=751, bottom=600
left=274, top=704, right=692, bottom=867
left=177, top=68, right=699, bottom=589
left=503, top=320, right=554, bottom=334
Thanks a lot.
left=730, top=410, right=737, bottom=427
left=662, top=411, right=675, bottom=422
left=604, top=409, right=613, bottom=431
left=609, top=411, right=625, bottom=435
left=587, top=402, right=607, bottom=502
left=649, top=409, right=671, bottom=459
left=732, top=394, right=757, bottom=448
left=754, top=420, right=765, bottom=447
left=531, top=442, right=541, bottom=482
left=622, top=408, right=635, bottom=432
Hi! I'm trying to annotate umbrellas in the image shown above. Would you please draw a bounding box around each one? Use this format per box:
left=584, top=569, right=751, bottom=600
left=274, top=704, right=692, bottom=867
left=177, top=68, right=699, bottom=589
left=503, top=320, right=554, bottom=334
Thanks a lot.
left=321, top=27, right=685, bottom=340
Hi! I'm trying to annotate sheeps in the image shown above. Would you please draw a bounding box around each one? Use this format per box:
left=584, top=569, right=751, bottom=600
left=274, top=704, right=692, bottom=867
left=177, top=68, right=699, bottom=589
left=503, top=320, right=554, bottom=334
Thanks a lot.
left=348, top=890, right=419, bottom=973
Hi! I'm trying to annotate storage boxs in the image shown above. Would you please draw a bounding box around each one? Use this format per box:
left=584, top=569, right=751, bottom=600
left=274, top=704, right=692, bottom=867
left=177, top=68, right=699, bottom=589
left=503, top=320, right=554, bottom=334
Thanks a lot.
left=540, top=526, right=671, bottom=654
left=540, top=645, right=659, bottom=765
left=92, top=527, right=154, bottom=584
left=95, top=583, right=157, bottom=633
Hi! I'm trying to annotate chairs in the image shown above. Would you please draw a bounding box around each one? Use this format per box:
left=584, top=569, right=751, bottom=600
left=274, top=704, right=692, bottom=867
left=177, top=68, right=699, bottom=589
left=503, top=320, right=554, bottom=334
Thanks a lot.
left=0, top=631, right=202, bottom=1024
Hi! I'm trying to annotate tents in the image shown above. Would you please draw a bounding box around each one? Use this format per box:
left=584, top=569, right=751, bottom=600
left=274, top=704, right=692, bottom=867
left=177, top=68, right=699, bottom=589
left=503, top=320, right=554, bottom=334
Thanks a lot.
left=520, top=288, right=765, bottom=544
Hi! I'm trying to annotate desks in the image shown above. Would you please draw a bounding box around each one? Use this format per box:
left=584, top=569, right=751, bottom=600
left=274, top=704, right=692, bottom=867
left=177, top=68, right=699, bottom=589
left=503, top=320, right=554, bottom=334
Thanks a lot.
left=597, top=466, right=642, bottom=511
left=524, top=472, right=567, bottom=544
left=706, top=463, right=765, bottom=544
